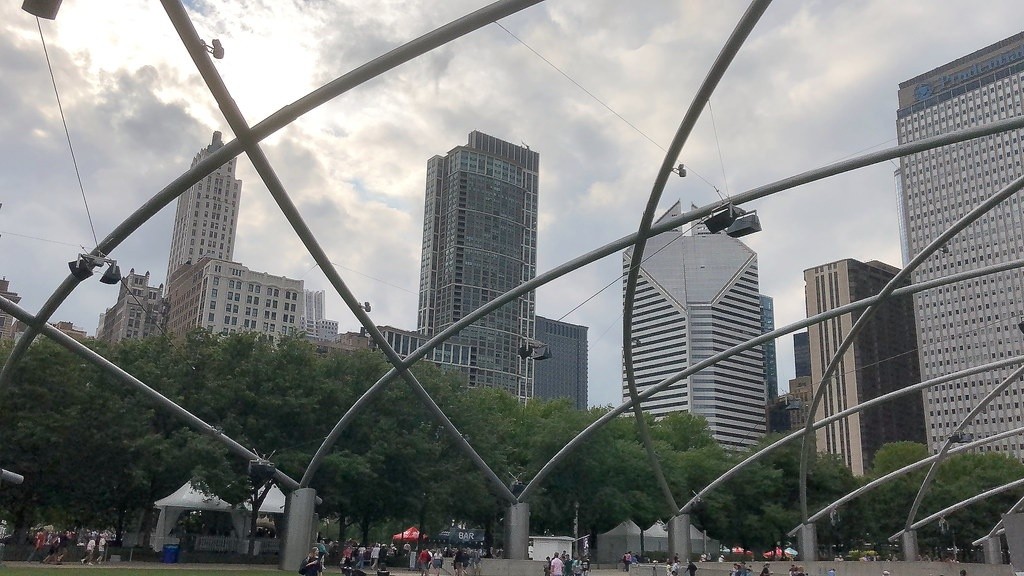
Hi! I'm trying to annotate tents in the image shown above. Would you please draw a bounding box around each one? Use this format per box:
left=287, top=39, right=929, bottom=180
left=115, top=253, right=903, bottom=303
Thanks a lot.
left=643, top=520, right=723, bottom=553
left=597, top=520, right=641, bottom=562
left=724, top=546, right=794, bottom=560
left=234, top=480, right=286, bottom=540
left=392, top=527, right=429, bottom=549
left=153, top=481, right=232, bottom=552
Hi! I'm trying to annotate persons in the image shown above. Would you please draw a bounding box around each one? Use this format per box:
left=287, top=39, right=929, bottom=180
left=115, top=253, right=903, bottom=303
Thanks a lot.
left=700, top=553, right=724, bottom=562
left=404, top=543, right=411, bottom=557
left=667, top=553, right=697, bottom=576
left=623, top=551, right=640, bottom=572
left=26, top=528, right=72, bottom=565
left=789, top=565, right=807, bottom=576
left=80, top=535, right=106, bottom=565
left=917, top=554, right=958, bottom=563
left=340, top=544, right=386, bottom=576
left=731, top=562, right=754, bottom=576
left=543, top=551, right=590, bottom=576
left=760, top=564, right=773, bottom=576
left=419, top=548, right=443, bottom=576
left=834, top=553, right=897, bottom=561
left=300, top=538, right=334, bottom=576
left=828, top=568, right=836, bottom=576
left=454, top=551, right=482, bottom=576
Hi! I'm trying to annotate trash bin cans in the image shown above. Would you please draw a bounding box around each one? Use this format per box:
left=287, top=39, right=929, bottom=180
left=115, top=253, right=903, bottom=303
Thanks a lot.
left=163, top=544, right=179, bottom=563
left=377, top=562, right=391, bottom=576
left=581, top=560, right=590, bottom=571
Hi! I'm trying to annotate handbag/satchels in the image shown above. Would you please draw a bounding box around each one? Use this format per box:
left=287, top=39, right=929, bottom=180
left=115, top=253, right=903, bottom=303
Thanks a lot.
left=299, top=557, right=308, bottom=575
